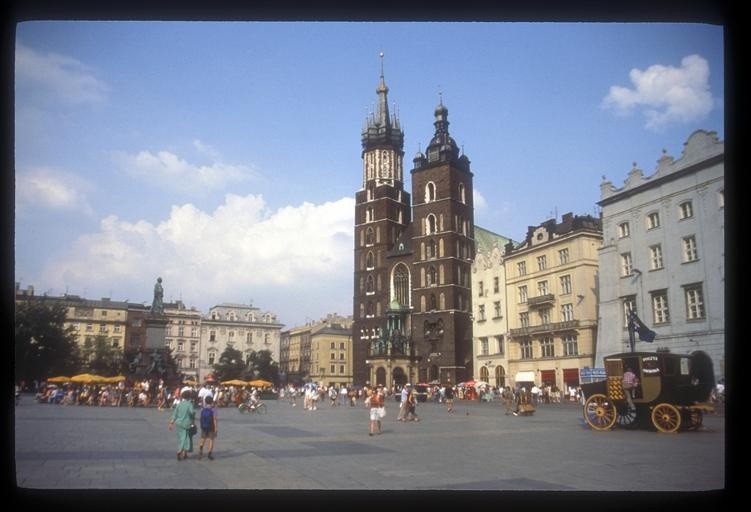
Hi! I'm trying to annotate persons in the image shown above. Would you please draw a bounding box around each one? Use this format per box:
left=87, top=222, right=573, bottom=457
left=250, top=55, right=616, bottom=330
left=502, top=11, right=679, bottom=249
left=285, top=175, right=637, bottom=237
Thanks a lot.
left=168, top=390, right=198, bottom=461
left=199, top=395, right=219, bottom=460
left=407, top=386, right=419, bottom=421
left=397, top=385, right=408, bottom=422
left=14, top=377, right=583, bottom=411
left=151, top=276, right=166, bottom=315
left=368, top=385, right=386, bottom=436
left=505, top=385, right=513, bottom=415
left=623, top=367, right=639, bottom=411
left=513, top=384, right=526, bottom=416
left=715, top=380, right=726, bottom=402
left=446, top=383, right=455, bottom=413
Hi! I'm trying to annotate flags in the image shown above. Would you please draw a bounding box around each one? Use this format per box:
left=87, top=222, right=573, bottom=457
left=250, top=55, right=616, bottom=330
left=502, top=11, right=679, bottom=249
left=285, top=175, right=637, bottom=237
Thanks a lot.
left=628, top=309, right=657, bottom=344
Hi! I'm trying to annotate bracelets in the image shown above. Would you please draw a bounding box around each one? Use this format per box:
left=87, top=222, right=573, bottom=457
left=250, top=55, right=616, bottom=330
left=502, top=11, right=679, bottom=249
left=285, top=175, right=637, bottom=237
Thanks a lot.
left=169, top=422, right=174, bottom=424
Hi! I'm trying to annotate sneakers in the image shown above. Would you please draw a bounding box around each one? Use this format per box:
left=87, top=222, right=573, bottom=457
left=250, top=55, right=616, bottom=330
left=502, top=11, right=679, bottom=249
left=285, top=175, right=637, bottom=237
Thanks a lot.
left=309, top=407, right=317, bottom=410
left=251, top=406, right=256, bottom=409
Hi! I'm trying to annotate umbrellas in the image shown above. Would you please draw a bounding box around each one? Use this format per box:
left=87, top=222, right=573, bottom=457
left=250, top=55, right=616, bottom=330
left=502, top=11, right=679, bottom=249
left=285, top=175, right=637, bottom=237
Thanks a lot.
left=47, top=373, right=128, bottom=383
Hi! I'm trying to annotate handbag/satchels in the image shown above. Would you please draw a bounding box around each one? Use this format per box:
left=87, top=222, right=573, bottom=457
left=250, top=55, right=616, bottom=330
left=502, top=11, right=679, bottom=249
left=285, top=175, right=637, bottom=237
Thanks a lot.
left=189, top=426, right=197, bottom=436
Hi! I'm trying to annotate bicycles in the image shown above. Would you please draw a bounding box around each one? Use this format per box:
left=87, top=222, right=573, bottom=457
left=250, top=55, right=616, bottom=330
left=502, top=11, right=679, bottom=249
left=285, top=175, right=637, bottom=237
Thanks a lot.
left=238, top=397, right=266, bottom=416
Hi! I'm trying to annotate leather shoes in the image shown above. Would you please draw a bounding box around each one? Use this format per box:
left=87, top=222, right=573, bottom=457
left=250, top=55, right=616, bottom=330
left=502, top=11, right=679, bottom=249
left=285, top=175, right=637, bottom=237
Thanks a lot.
left=177, top=452, right=214, bottom=461
